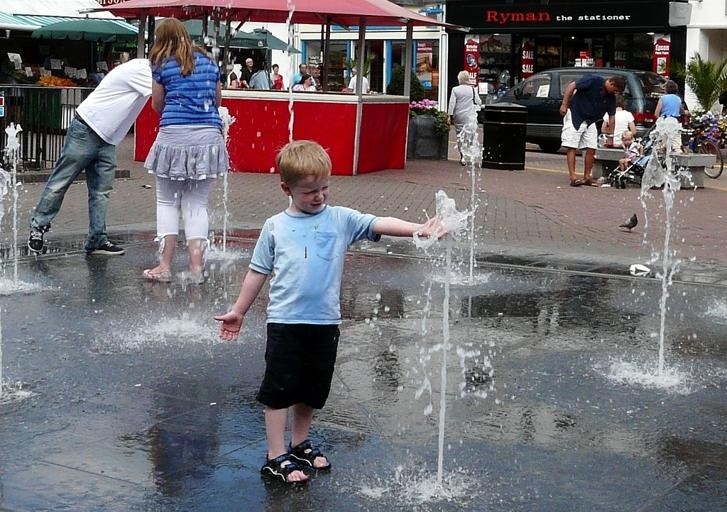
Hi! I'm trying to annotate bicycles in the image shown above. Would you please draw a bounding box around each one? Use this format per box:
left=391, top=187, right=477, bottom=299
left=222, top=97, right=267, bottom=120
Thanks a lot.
left=679, top=115, right=724, bottom=178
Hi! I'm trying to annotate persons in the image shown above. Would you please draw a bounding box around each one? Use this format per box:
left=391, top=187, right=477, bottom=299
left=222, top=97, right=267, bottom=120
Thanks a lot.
left=142, top=17, right=231, bottom=286
left=222, top=54, right=373, bottom=94
left=558, top=76, right=685, bottom=187
left=211, top=139, right=444, bottom=488
left=27, top=57, right=154, bottom=255
left=448, top=70, right=483, bottom=167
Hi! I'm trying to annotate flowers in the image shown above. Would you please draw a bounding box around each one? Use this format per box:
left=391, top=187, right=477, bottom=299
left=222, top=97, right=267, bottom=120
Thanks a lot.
left=408, top=96, right=450, bottom=133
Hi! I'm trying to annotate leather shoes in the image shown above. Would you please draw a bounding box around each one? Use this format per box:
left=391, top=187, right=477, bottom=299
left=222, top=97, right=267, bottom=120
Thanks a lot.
left=176, top=271, right=205, bottom=284
left=142, top=269, right=172, bottom=283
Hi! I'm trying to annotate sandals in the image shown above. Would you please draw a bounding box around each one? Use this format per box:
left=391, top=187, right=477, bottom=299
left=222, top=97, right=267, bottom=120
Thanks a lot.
left=287, top=439, right=332, bottom=470
left=260, top=454, right=308, bottom=486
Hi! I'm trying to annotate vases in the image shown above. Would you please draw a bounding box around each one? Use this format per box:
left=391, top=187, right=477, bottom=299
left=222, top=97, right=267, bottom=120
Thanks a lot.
left=407, top=116, right=447, bottom=159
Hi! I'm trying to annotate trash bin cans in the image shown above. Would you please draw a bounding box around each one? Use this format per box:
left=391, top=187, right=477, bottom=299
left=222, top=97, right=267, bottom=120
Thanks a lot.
left=476, top=102, right=529, bottom=169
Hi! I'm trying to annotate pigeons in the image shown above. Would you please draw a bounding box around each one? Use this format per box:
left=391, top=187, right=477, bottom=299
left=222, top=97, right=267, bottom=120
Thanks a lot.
left=618, top=212, right=639, bottom=233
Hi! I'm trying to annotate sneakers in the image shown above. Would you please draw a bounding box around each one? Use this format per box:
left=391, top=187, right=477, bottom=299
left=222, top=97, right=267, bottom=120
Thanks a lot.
left=90, top=241, right=126, bottom=255
left=27, top=224, right=43, bottom=252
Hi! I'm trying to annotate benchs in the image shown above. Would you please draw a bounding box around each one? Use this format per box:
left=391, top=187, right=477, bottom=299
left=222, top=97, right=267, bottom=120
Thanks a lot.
left=592, top=146, right=717, bottom=191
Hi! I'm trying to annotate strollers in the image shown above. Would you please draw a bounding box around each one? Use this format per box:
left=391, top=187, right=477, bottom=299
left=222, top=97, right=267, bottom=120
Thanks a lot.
left=605, top=124, right=666, bottom=190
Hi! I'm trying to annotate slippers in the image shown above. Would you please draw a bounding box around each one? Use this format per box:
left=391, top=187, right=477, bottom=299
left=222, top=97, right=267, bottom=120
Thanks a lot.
left=583, top=178, right=598, bottom=187
left=569, top=179, right=583, bottom=186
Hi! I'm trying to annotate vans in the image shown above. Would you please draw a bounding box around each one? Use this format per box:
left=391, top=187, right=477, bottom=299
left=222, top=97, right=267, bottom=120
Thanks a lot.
left=484, top=66, right=668, bottom=154
